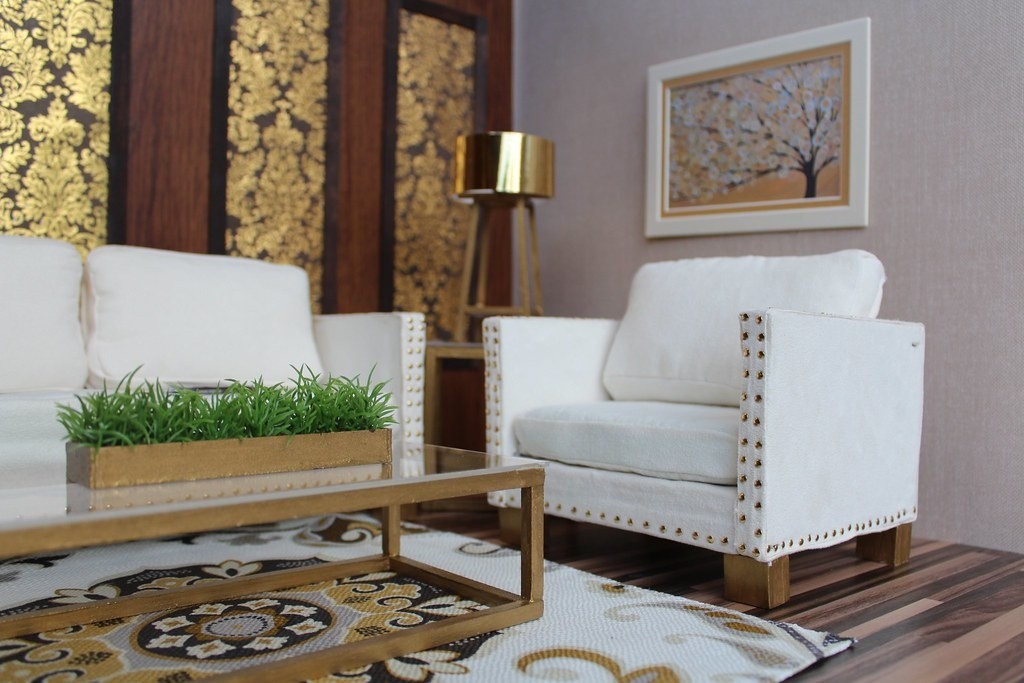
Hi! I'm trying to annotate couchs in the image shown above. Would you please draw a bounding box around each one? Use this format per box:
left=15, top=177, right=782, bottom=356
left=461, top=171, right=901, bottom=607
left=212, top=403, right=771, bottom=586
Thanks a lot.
left=479, top=247, right=928, bottom=610
left=0, top=236, right=427, bottom=442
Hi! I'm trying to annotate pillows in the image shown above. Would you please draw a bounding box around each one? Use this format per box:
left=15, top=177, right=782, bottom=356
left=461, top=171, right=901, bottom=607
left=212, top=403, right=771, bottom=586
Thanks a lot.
left=83, top=244, right=323, bottom=391
left=602, top=249, right=887, bottom=406
left=0, top=236, right=88, bottom=391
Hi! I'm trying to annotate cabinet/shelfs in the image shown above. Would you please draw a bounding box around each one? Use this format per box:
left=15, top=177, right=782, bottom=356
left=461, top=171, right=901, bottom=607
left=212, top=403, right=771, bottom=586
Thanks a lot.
left=414, top=340, right=498, bottom=522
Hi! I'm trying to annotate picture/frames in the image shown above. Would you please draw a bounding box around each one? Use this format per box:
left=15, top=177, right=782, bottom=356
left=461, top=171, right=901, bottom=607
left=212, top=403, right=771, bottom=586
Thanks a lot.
left=642, top=17, right=871, bottom=241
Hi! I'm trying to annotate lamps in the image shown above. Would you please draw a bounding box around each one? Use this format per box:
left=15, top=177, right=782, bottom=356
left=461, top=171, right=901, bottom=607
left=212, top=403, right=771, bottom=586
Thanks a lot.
left=448, top=130, right=556, bottom=342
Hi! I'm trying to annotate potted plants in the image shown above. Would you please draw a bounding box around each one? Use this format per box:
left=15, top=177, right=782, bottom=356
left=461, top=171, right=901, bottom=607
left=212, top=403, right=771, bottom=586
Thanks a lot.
left=58, top=361, right=394, bottom=489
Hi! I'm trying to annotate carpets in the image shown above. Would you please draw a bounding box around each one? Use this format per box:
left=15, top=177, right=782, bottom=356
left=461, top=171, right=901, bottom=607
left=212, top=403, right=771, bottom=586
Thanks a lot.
left=1, top=511, right=857, bottom=683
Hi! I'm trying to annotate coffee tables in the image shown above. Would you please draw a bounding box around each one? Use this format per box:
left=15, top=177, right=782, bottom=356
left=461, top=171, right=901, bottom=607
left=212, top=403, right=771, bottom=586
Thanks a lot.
left=0, top=443, right=544, bottom=683
